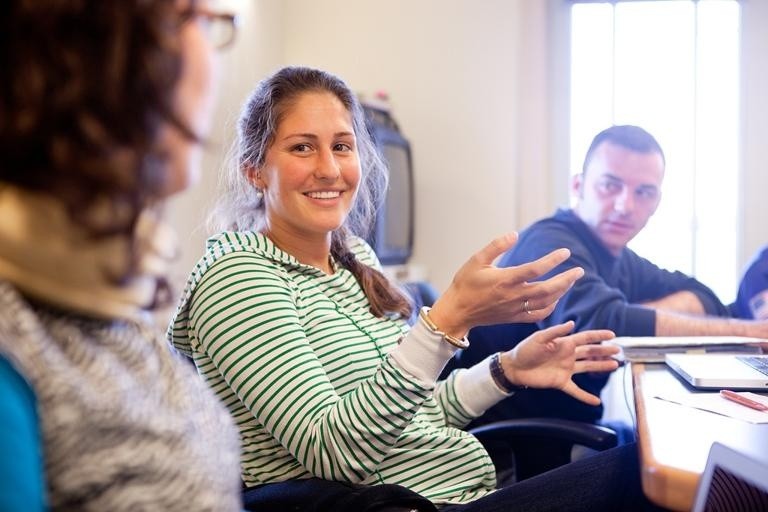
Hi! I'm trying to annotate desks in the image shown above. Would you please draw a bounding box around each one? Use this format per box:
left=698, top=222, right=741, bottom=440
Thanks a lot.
left=633, top=358, right=768, bottom=512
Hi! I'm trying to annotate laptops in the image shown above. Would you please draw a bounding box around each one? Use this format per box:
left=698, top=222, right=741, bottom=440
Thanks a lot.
left=665, top=352, right=768, bottom=389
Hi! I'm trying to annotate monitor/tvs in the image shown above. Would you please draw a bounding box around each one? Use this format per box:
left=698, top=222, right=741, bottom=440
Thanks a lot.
left=362, top=127, right=415, bottom=268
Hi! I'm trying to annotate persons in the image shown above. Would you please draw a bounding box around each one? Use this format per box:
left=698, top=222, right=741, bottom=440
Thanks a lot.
left=456, top=125, right=767, bottom=491
left=166, top=66, right=663, bottom=512
left=0, top=0, right=248, bottom=511
left=732, top=247, right=768, bottom=323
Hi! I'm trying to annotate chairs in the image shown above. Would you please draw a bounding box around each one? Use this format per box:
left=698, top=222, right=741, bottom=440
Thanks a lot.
left=241, top=416, right=619, bottom=512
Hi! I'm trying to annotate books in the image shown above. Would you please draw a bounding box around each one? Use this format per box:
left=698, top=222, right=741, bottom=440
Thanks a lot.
left=602, top=333, right=768, bottom=364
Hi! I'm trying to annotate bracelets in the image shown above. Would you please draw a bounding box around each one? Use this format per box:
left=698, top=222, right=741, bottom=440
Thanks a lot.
left=419, top=305, right=469, bottom=349
left=490, top=351, right=527, bottom=393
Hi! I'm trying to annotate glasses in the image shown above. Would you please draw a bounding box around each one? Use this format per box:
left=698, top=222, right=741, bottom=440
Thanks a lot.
left=180, top=7, right=239, bottom=51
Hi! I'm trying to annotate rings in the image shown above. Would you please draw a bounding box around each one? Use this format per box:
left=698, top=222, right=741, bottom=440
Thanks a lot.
left=523, top=300, right=531, bottom=314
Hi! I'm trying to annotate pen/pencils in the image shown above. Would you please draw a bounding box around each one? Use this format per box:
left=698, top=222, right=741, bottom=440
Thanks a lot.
left=720, top=390, right=768, bottom=412
